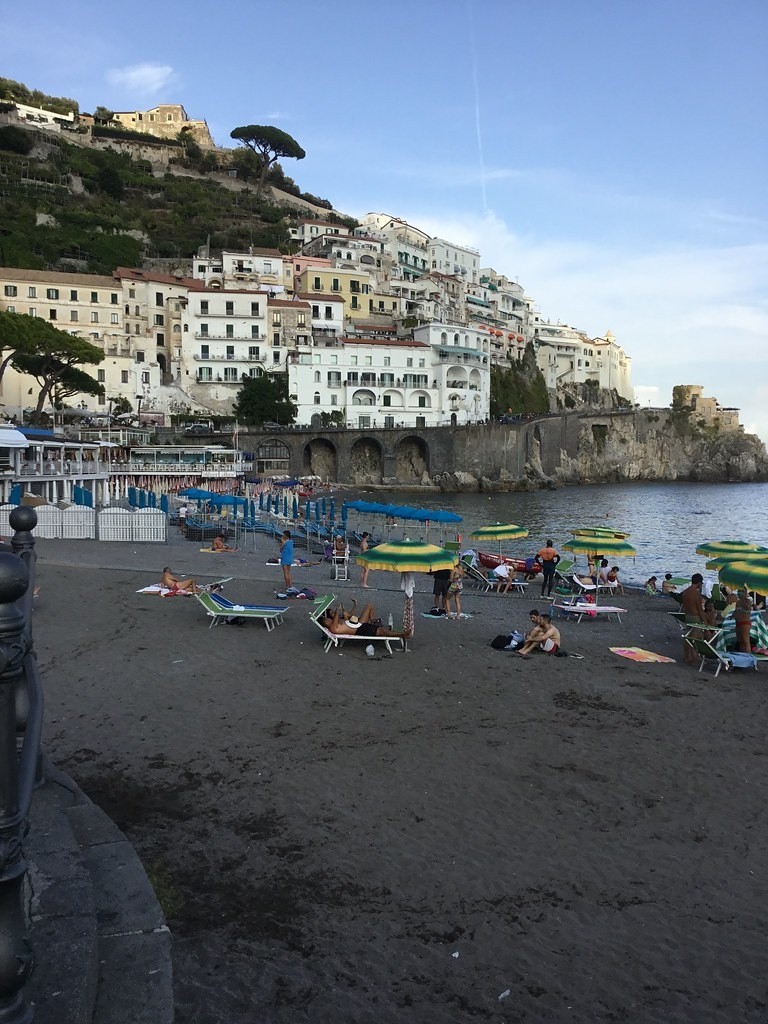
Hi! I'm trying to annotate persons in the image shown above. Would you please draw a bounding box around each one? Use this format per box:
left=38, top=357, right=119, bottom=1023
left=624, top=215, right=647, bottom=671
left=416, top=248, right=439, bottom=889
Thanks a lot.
left=427, top=563, right=464, bottom=620
left=179, top=503, right=187, bottom=529
left=335, top=536, right=346, bottom=555
left=318, top=598, right=412, bottom=637
left=212, top=534, right=238, bottom=552
left=161, top=567, right=203, bottom=592
left=644, top=574, right=676, bottom=593
left=359, top=532, right=370, bottom=587
left=576, top=554, right=624, bottom=596
left=678, top=573, right=766, bottom=664
left=280, top=530, right=294, bottom=587
left=535, top=540, right=560, bottom=597
left=466, top=411, right=551, bottom=425
left=493, top=563, right=517, bottom=595
left=516, top=609, right=560, bottom=656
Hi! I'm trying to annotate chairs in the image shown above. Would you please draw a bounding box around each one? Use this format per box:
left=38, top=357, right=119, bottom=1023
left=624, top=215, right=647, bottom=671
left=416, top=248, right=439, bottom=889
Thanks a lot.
left=170, top=497, right=768, bottom=678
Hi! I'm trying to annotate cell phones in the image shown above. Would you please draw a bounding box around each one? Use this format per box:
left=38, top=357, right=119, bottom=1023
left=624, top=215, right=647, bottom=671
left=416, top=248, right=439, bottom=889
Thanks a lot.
left=351, top=599, right=356, bottom=602
left=341, top=603, right=344, bottom=611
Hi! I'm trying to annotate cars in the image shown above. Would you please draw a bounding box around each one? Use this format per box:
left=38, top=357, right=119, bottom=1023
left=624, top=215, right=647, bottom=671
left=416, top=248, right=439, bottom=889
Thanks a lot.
left=185, top=423, right=208, bottom=433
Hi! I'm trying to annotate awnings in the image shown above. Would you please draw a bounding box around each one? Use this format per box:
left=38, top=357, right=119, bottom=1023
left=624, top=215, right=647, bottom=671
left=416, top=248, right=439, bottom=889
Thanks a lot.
left=434, top=345, right=489, bottom=359
left=468, top=297, right=491, bottom=307
left=404, top=268, right=420, bottom=277
left=480, top=326, right=524, bottom=342
left=454, top=265, right=467, bottom=274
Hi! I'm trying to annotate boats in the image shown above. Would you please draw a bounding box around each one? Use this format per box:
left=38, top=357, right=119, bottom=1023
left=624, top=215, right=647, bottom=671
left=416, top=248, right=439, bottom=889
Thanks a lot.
left=478, top=550, right=543, bottom=573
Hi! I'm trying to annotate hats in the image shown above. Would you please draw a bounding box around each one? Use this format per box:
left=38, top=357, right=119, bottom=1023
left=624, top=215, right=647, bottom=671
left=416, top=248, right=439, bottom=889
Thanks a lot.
left=345, top=615, right=362, bottom=629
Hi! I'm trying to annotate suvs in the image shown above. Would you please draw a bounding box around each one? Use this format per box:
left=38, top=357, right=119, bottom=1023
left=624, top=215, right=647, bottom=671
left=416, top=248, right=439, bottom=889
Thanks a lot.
left=262, top=421, right=285, bottom=432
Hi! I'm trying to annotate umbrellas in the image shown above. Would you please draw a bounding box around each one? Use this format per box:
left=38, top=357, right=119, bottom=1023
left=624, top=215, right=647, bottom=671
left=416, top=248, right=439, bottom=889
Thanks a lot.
left=356, top=538, right=459, bottom=652
left=562, top=525, right=637, bottom=604
left=74, top=485, right=92, bottom=507
left=696, top=540, right=768, bottom=606
left=42, top=407, right=97, bottom=417
left=8, top=483, right=21, bottom=504
left=103, top=477, right=463, bottom=551
left=468, top=521, right=529, bottom=564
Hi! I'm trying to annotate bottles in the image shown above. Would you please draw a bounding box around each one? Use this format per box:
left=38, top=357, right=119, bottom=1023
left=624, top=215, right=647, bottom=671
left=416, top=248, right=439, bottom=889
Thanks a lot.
left=388, top=614, right=393, bottom=631
